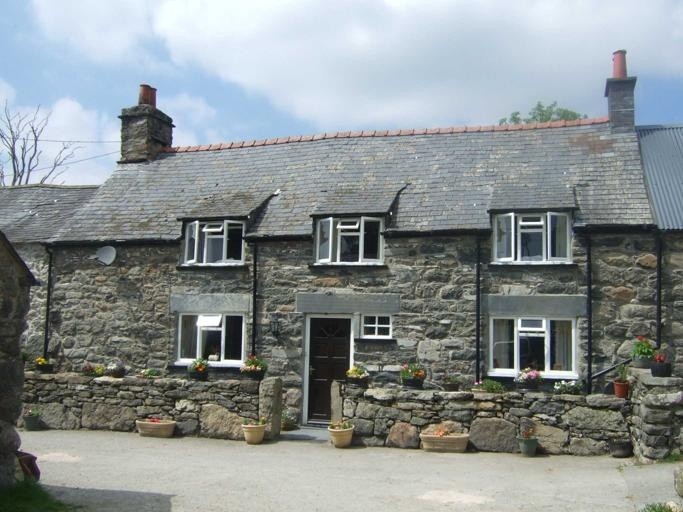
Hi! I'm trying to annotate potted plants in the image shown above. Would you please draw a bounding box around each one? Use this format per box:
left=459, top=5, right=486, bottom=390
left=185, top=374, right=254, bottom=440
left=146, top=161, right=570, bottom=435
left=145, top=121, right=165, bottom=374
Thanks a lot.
left=327, top=417, right=355, bottom=448
left=240, top=414, right=270, bottom=443
left=608, top=359, right=631, bottom=396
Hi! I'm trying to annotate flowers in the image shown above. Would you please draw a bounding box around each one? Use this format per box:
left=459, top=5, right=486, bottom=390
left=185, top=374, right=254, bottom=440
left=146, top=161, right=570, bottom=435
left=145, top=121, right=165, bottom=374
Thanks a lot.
left=631, top=335, right=671, bottom=366
left=32, top=354, right=49, bottom=366
left=239, top=352, right=268, bottom=373
left=431, top=425, right=452, bottom=436
left=521, top=428, right=531, bottom=438
left=345, top=362, right=369, bottom=379
left=79, top=359, right=105, bottom=376
left=21, top=404, right=43, bottom=430
left=400, top=358, right=584, bottom=396
left=188, top=357, right=209, bottom=372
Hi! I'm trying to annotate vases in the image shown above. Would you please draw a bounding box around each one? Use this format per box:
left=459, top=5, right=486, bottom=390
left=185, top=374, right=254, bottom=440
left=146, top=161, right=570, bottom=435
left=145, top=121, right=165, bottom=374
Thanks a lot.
left=516, top=436, right=539, bottom=456
left=650, top=362, right=673, bottom=377
left=419, top=430, right=471, bottom=452
left=37, top=364, right=53, bottom=375
left=137, top=419, right=176, bottom=437
left=347, top=377, right=368, bottom=389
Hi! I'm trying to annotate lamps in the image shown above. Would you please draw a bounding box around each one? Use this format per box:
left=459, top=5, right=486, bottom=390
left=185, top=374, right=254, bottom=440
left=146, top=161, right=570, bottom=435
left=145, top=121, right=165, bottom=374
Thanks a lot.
left=267, top=311, right=283, bottom=340
